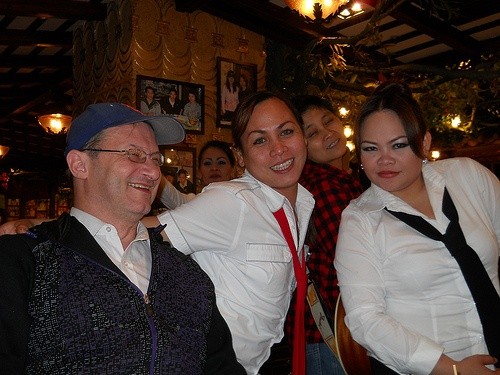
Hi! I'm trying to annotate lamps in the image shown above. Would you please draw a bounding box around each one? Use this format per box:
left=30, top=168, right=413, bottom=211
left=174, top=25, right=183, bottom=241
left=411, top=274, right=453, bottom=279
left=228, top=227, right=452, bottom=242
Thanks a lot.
left=0, top=144, right=10, bottom=160
left=283, top=0, right=352, bottom=25
left=36, top=110, right=74, bottom=137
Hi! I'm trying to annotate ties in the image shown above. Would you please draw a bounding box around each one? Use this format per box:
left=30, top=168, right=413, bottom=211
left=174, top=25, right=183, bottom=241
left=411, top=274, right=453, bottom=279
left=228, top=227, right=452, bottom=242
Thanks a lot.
left=384, top=187, right=500, bottom=368
left=272, top=207, right=307, bottom=375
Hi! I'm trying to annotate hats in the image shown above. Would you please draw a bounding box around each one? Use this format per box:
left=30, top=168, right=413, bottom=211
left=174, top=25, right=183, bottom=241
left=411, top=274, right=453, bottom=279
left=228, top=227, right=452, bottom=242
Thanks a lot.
left=65, top=102, right=185, bottom=154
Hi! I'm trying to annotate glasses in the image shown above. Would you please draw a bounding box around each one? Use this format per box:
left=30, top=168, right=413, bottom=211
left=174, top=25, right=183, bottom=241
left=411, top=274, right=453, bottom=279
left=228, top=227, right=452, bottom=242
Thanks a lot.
left=80, top=148, right=165, bottom=167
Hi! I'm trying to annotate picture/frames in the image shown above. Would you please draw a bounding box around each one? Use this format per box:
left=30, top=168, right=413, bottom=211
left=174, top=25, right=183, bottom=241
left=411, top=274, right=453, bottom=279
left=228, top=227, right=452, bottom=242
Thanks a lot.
left=158, top=145, right=199, bottom=194
left=216, top=56, right=258, bottom=129
left=136, top=74, right=206, bottom=135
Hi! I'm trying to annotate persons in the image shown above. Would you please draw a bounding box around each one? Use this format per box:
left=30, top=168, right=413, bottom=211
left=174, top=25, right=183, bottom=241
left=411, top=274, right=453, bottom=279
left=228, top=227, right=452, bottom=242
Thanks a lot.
left=140, top=86, right=160, bottom=113
left=221, top=70, right=252, bottom=115
left=0, top=81, right=500, bottom=375
left=160, top=88, right=183, bottom=115
left=183, top=92, right=201, bottom=122
left=164, top=171, right=175, bottom=185
left=172, top=169, right=194, bottom=194
